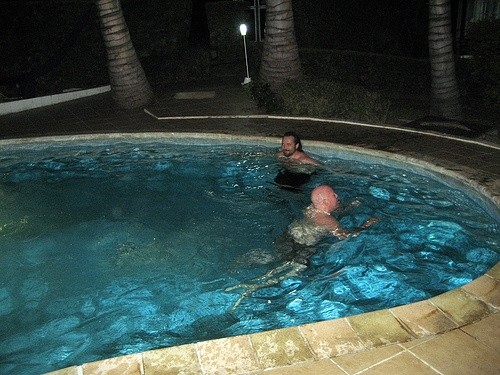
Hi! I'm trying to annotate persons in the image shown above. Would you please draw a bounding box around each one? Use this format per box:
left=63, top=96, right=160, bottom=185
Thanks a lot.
left=275, top=185, right=380, bottom=267
left=266, top=132, right=321, bottom=193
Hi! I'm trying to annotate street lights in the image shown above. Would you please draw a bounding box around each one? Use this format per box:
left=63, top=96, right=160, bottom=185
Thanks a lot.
left=239, top=23, right=252, bottom=84
